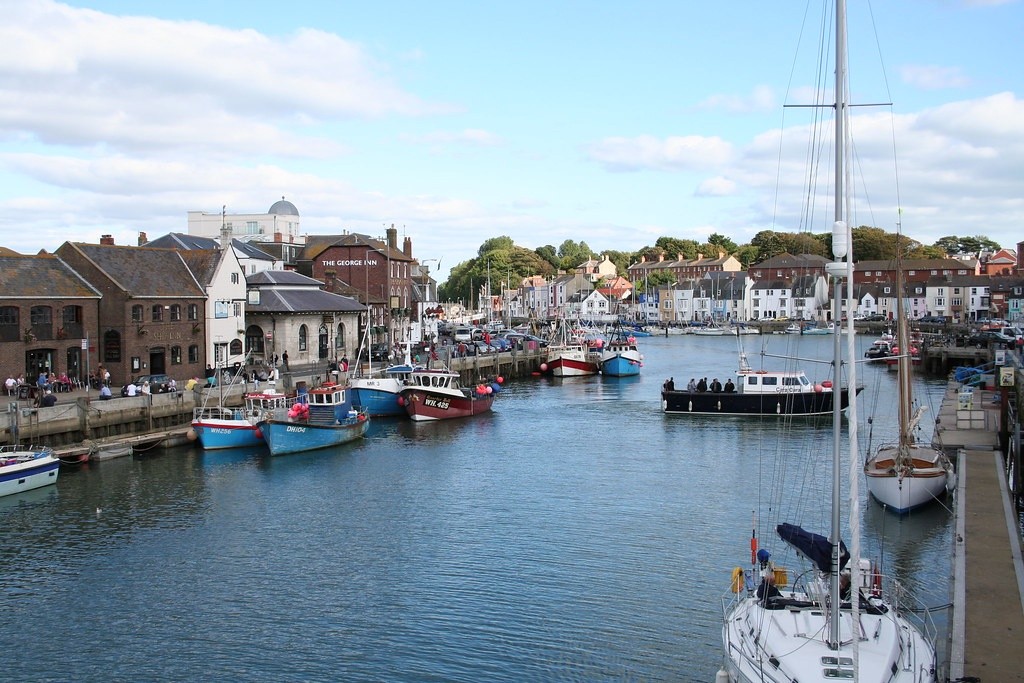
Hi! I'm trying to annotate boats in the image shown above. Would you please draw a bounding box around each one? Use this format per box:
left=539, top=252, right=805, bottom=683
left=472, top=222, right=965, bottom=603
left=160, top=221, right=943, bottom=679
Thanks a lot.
left=0, top=444, right=60, bottom=498
left=346, top=306, right=497, bottom=423
left=865, top=330, right=932, bottom=371
left=191, top=350, right=371, bottom=456
left=661, top=325, right=864, bottom=415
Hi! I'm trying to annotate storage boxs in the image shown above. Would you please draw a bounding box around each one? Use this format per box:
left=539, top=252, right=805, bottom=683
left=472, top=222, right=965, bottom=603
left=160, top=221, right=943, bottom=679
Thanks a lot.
left=347, top=410, right=357, bottom=422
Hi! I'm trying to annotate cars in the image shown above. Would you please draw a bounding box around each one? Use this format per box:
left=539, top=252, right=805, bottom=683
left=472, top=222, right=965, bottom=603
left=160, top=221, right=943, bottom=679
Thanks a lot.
left=366, top=342, right=389, bottom=362
left=969, top=332, right=1015, bottom=350
left=977, top=316, right=1009, bottom=326
left=916, top=316, right=947, bottom=323
left=757, top=312, right=888, bottom=323
left=438, top=320, right=549, bottom=356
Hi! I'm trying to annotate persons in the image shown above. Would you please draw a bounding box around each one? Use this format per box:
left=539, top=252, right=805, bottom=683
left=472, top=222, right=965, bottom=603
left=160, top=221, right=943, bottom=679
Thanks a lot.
left=341, top=339, right=464, bottom=374
left=127, top=350, right=289, bottom=406
left=663, top=376, right=735, bottom=393
left=1018, top=337, right=1023, bottom=354
left=4, top=363, right=111, bottom=408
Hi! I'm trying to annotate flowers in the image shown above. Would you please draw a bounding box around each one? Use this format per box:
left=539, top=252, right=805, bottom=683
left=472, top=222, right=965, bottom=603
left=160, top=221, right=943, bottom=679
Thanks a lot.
left=139, top=329, right=149, bottom=336
left=25, top=332, right=37, bottom=343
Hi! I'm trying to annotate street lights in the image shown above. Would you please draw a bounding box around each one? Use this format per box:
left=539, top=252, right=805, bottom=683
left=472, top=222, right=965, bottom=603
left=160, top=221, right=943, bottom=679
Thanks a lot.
left=420, top=258, right=437, bottom=341
left=365, top=248, right=385, bottom=378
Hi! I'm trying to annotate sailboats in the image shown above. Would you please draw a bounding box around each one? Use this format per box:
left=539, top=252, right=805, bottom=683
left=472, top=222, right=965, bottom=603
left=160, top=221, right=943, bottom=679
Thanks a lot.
left=479, top=257, right=858, bottom=376
left=717, top=12, right=942, bottom=683
left=861, top=207, right=958, bottom=515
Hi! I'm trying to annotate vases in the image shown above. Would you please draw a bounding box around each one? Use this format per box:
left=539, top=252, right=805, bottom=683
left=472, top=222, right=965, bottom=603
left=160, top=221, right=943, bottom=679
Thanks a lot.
left=58, top=328, right=68, bottom=339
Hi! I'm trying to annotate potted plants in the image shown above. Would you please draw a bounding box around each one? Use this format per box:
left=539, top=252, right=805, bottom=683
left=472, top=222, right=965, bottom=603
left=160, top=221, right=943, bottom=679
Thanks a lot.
left=238, top=330, right=246, bottom=335
left=196, top=327, right=201, bottom=332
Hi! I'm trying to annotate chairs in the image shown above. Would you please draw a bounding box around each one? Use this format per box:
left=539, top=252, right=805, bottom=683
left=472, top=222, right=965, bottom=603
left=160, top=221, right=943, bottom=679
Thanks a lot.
left=4, top=380, right=84, bottom=396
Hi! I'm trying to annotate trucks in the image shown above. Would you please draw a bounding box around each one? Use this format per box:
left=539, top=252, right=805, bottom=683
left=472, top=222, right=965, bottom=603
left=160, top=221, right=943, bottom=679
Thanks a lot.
left=982, top=327, right=1024, bottom=341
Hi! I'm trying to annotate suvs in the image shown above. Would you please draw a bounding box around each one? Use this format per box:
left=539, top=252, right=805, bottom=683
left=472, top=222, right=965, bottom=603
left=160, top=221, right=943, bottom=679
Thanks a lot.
left=120, top=374, right=170, bottom=397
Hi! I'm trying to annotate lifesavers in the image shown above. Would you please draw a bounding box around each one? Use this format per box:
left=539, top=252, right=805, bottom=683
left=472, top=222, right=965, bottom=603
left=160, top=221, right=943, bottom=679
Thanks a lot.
left=756, top=371, right=767, bottom=374
left=732, top=567, right=743, bottom=594
